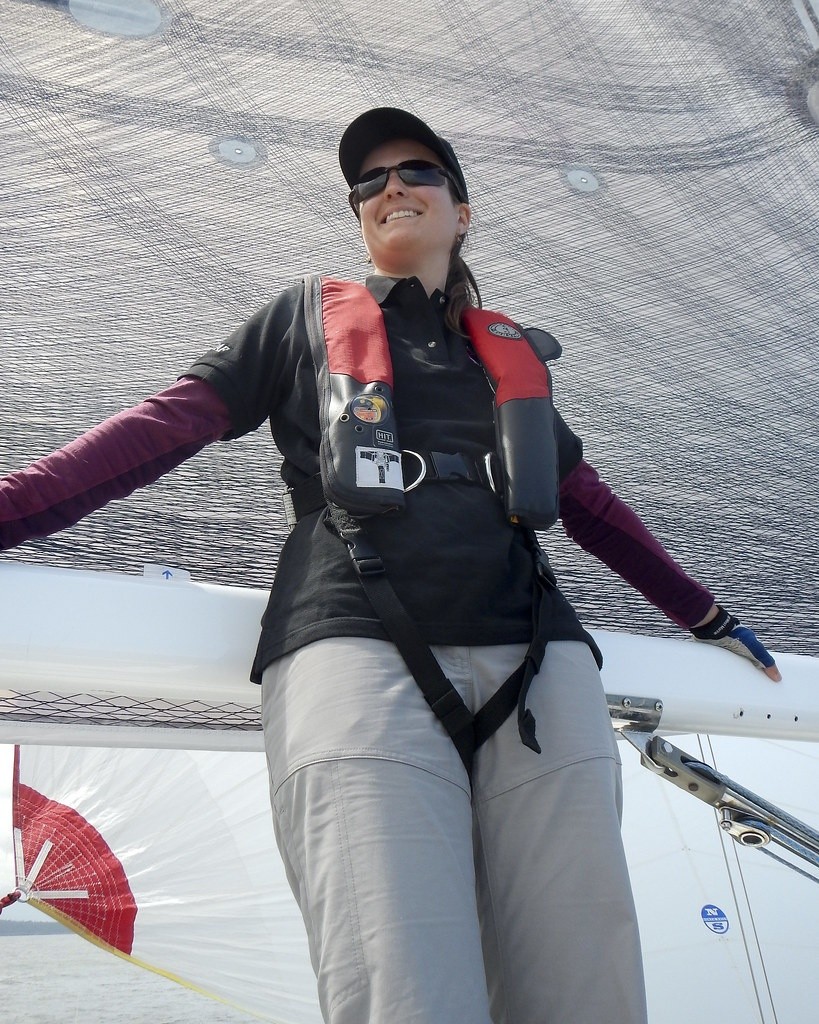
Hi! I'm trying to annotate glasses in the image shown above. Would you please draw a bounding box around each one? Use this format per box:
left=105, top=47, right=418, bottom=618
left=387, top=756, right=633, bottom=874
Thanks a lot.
left=347, top=159, right=463, bottom=220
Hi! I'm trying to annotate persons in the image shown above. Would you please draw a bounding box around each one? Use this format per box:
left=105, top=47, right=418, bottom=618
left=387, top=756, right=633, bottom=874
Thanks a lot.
left=0, top=105, right=780, bottom=1023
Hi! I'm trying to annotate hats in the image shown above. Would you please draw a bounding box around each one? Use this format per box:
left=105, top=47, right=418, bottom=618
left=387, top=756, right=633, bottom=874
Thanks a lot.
left=339, top=107, right=467, bottom=201
